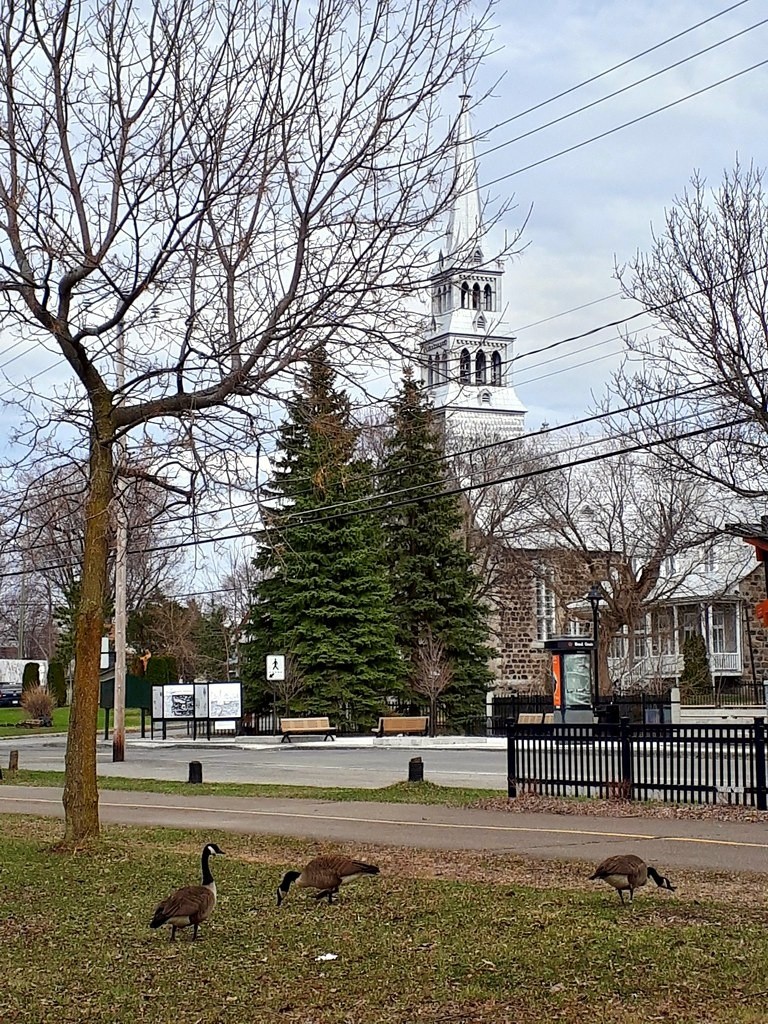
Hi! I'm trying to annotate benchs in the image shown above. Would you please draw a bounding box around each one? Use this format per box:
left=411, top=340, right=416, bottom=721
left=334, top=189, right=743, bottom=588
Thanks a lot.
left=371, top=716, right=429, bottom=738
left=513, top=713, right=543, bottom=736
left=543, top=713, right=554, bottom=735
left=280, top=717, right=336, bottom=743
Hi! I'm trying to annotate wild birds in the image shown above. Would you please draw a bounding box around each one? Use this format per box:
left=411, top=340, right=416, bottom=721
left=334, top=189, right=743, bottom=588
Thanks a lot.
left=151, top=842, right=226, bottom=944
left=589, top=855, right=676, bottom=906
left=276, top=855, right=379, bottom=907
